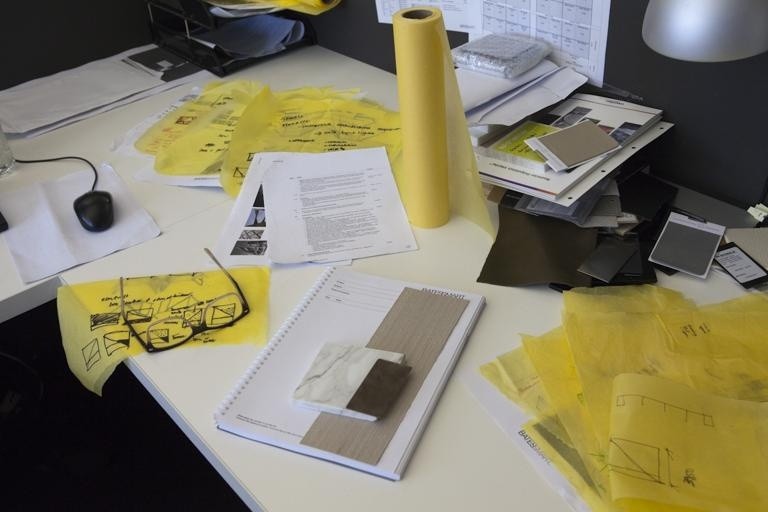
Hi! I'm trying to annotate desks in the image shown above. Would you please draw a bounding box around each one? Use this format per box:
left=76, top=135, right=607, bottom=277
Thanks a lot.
left=0, top=193, right=767, bottom=512
left=0, top=39, right=396, bottom=346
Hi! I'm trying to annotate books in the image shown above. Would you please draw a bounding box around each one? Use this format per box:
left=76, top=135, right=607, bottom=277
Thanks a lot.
left=214, top=264, right=487, bottom=480
left=291, top=340, right=406, bottom=423
left=455, top=57, right=674, bottom=223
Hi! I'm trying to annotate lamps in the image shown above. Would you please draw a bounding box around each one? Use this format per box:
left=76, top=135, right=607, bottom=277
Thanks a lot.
left=640, top=0, right=768, bottom=277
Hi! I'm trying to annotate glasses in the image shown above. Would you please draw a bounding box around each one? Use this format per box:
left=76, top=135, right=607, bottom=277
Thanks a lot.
left=116, top=247, right=251, bottom=355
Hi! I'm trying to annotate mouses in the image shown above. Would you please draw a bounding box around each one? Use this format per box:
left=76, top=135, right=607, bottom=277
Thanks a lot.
left=73, top=190, right=114, bottom=232
